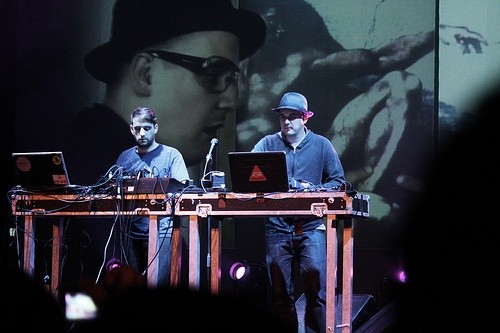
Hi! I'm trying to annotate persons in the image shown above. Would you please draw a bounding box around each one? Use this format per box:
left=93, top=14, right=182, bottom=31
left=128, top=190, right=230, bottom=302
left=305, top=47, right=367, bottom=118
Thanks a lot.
left=250, top=92, right=346, bottom=333
left=31, top=0, right=268, bottom=271
left=112, top=107, right=191, bottom=291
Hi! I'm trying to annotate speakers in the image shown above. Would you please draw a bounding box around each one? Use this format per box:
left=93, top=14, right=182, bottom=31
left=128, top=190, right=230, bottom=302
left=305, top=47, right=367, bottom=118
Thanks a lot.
left=317, top=293, right=419, bottom=333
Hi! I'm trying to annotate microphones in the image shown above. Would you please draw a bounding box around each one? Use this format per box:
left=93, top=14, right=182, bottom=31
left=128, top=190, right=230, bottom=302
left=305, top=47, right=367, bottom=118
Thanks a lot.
left=206, top=138, right=219, bottom=162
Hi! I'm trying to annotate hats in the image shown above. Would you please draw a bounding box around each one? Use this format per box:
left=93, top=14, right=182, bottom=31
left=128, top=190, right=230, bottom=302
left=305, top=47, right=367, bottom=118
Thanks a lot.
left=85, top=0, right=267, bottom=82
left=271, top=92, right=314, bottom=118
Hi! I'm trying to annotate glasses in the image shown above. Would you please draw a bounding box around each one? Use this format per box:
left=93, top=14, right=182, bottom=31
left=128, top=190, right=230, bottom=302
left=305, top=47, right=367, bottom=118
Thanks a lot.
left=136, top=48, right=240, bottom=94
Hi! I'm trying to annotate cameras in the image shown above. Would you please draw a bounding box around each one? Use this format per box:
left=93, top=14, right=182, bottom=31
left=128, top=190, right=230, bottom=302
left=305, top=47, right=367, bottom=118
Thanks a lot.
left=64, top=291, right=99, bottom=320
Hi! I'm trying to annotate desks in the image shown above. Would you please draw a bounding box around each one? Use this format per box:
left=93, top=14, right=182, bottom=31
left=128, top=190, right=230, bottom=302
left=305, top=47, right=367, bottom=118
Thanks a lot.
left=174, top=189, right=370, bottom=333
left=10, top=194, right=185, bottom=299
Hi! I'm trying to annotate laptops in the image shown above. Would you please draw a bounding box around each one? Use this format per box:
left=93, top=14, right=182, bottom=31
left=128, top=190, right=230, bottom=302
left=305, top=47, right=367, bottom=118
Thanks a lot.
left=228, top=151, right=305, bottom=193
left=12, top=151, right=82, bottom=188
